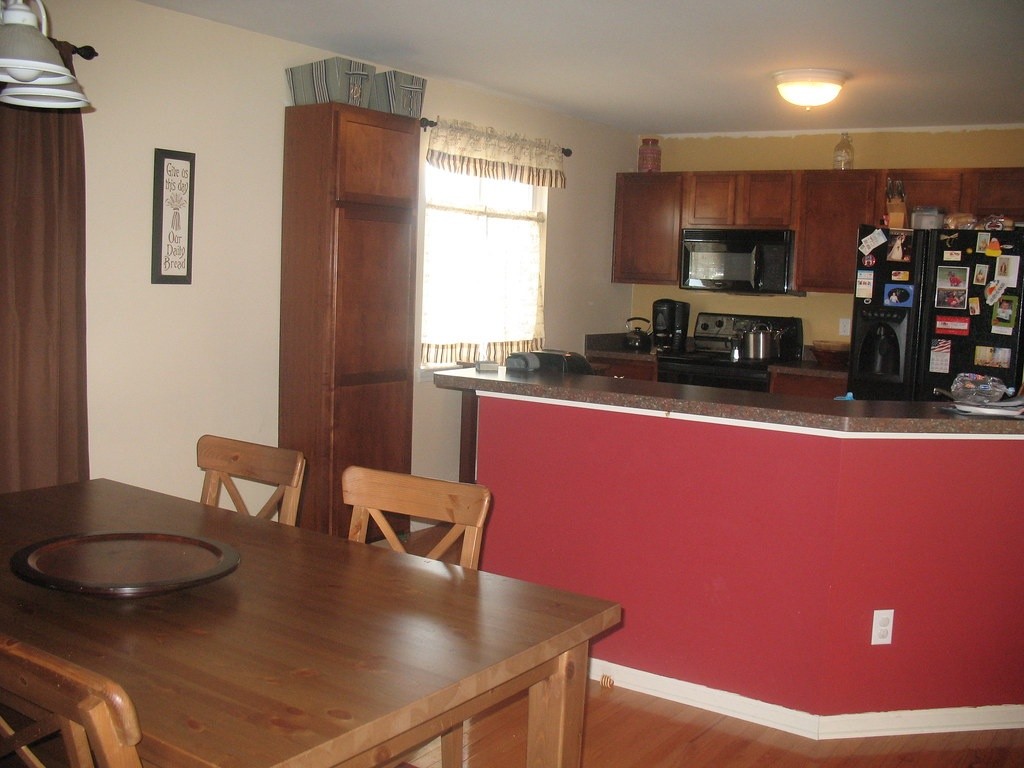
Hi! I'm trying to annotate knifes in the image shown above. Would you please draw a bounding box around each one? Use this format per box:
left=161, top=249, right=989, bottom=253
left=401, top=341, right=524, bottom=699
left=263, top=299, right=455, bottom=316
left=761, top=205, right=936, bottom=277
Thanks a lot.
left=884, top=177, right=906, bottom=203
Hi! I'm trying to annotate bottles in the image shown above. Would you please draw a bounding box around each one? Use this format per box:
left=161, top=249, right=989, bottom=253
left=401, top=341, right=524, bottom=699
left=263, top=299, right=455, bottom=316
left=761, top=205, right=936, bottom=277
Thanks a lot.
left=637, top=138, right=662, bottom=172
left=832, top=131, right=854, bottom=170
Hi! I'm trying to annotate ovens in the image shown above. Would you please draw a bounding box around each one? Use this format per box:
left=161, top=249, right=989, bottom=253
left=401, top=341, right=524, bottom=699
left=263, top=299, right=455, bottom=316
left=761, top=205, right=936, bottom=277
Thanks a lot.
left=656, top=358, right=770, bottom=395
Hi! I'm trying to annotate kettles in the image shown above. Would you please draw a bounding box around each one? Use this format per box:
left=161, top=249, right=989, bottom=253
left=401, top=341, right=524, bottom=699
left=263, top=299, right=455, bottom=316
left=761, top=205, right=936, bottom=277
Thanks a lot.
left=623, top=316, right=652, bottom=350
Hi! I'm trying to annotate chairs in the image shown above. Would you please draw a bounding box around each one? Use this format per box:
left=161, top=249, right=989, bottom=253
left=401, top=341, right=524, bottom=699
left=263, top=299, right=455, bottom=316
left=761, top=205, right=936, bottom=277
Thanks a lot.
left=198, top=434, right=306, bottom=529
left=342, top=466, right=491, bottom=574
left=0, top=631, right=144, bottom=768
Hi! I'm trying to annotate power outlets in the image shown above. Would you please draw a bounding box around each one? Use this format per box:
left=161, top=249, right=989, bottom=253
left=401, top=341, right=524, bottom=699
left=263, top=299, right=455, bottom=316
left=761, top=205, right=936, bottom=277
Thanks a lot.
left=871, top=608, right=894, bottom=645
left=839, top=317, right=851, bottom=336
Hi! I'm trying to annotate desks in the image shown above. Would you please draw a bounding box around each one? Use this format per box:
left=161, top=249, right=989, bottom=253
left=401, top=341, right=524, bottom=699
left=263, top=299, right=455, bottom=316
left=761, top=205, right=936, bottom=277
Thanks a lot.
left=0, top=478, right=620, bottom=768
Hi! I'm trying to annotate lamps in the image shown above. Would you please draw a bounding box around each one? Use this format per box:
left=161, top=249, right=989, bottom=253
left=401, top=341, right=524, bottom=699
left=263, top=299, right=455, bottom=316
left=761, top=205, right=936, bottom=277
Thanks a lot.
left=0, top=0, right=101, bottom=110
left=771, top=69, right=851, bottom=110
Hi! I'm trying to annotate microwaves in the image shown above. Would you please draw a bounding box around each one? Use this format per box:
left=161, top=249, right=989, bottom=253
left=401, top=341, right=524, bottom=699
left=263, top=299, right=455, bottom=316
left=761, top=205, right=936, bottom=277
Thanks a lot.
left=679, top=228, right=807, bottom=299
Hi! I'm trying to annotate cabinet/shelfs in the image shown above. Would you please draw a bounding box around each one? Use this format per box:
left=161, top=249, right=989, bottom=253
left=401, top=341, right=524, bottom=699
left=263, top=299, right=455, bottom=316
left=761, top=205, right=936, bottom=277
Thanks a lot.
left=278, top=109, right=423, bottom=544
left=683, top=169, right=793, bottom=229
left=612, top=170, right=683, bottom=285
left=793, top=168, right=877, bottom=293
left=878, top=166, right=1024, bottom=226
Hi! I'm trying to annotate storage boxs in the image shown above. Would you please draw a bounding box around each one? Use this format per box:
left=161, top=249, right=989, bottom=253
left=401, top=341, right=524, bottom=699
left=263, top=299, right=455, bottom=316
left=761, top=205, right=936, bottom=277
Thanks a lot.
left=369, top=70, right=427, bottom=119
left=285, top=56, right=376, bottom=105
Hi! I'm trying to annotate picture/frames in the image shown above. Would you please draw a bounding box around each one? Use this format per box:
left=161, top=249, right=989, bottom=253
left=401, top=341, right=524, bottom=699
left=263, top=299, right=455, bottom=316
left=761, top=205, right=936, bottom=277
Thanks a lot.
left=150, top=148, right=197, bottom=284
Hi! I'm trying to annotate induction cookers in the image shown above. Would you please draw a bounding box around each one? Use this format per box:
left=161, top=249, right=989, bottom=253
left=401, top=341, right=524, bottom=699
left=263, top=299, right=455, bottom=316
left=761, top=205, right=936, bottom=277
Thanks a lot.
left=656, top=311, right=804, bottom=368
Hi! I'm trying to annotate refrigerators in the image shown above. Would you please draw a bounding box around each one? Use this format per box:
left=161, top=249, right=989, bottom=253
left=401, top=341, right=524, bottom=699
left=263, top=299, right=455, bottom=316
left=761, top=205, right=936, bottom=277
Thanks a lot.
left=846, top=223, right=1024, bottom=401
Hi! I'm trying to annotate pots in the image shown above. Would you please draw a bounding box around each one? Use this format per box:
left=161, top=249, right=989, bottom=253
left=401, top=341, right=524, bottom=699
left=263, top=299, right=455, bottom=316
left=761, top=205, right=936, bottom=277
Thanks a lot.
left=736, top=324, right=784, bottom=358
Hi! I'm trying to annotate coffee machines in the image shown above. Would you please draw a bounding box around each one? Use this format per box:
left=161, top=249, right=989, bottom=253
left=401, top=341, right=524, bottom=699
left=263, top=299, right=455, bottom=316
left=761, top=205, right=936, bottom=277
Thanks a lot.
left=651, top=299, right=690, bottom=353
